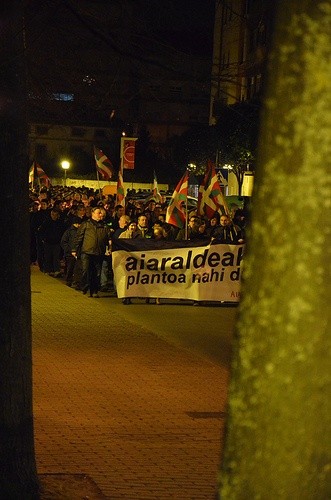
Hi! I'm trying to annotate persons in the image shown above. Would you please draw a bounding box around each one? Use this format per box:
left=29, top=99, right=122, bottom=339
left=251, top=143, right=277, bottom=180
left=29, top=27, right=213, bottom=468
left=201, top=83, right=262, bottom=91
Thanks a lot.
left=26, top=185, right=251, bottom=298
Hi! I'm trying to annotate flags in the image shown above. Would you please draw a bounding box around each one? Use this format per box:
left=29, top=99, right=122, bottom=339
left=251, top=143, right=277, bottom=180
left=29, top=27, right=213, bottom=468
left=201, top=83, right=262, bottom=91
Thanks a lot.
left=117, top=171, right=125, bottom=205
left=28, top=164, right=34, bottom=183
left=165, top=170, right=188, bottom=229
left=36, top=161, right=49, bottom=179
left=197, top=160, right=228, bottom=222
left=145, top=176, right=166, bottom=204
left=94, top=144, right=115, bottom=181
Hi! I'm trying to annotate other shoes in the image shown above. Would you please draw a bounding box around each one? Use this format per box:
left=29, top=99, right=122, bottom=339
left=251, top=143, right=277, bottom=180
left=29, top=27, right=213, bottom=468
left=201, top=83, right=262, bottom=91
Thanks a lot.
left=122, top=298, right=132, bottom=305
left=145, top=298, right=161, bottom=305
left=48, top=272, right=100, bottom=298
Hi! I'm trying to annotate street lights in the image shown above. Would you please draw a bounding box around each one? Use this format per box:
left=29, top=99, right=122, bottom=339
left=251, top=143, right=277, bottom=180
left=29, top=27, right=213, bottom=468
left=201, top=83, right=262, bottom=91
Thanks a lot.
left=61, top=161, right=70, bottom=187
